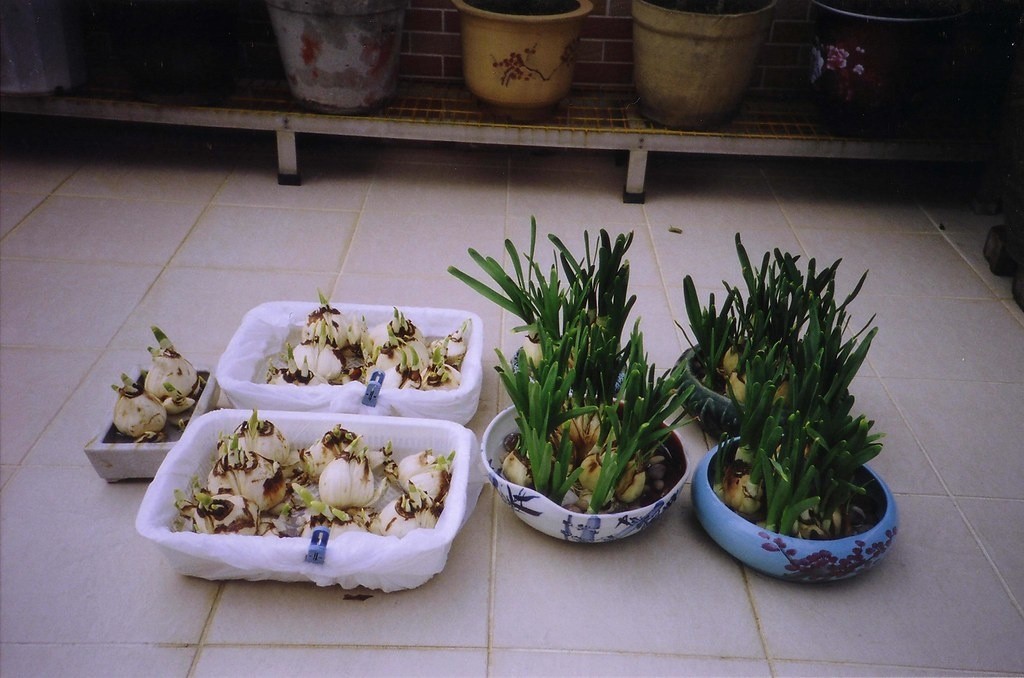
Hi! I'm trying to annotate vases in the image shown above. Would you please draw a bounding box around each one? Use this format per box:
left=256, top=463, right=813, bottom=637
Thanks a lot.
left=452, top=0, right=594, bottom=123
left=632, top=0, right=778, bottom=128
left=806, top=0, right=932, bottom=137
left=267, top=0, right=409, bottom=116
left=0, top=0, right=79, bottom=94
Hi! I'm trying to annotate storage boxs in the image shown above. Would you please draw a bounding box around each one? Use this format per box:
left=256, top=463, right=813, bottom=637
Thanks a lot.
left=212, top=300, right=485, bottom=427
left=135, top=408, right=479, bottom=596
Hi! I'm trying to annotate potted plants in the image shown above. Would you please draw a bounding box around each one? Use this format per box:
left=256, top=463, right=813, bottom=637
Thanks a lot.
left=446, top=217, right=900, bottom=583
left=83, top=326, right=220, bottom=481
